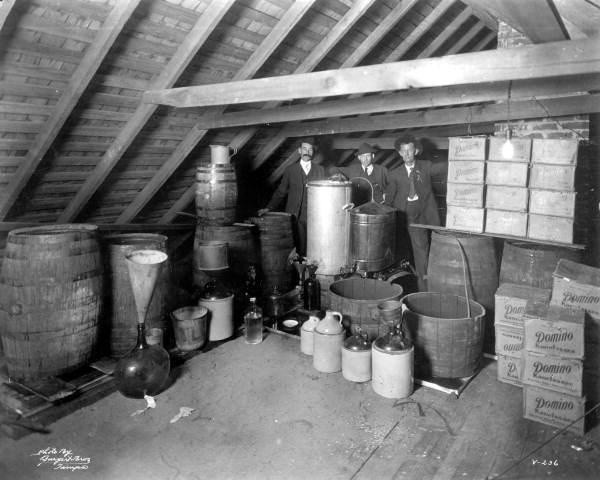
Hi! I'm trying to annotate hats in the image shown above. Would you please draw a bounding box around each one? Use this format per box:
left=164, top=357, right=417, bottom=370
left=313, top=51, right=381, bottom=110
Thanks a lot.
left=354, top=142, right=379, bottom=156
left=296, top=137, right=320, bottom=152
left=394, top=134, right=424, bottom=156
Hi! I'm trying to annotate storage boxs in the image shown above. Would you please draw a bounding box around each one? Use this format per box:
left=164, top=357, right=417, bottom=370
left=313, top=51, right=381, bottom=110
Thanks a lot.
left=493, top=258, right=600, bottom=437
left=443, top=135, right=579, bottom=246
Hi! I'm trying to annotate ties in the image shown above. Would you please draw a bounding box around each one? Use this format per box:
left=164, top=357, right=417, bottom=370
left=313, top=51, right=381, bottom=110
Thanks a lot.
left=364, top=167, right=368, bottom=177
left=408, top=167, right=417, bottom=199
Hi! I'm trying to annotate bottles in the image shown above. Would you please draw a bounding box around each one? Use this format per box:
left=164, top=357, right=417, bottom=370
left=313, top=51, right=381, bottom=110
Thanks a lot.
left=244, top=297, right=263, bottom=345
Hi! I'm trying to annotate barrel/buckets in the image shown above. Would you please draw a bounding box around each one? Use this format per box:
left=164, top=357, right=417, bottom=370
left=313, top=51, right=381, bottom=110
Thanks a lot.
left=171, top=307, right=208, bottom=352
left=193, top=224, right=256, bottom=317
left=254, top=211, right=295, bottom=274
left=330, top=279, right=403, bottom=331
left=196, top=163, right=238, bottom=226
left=0, top=223, right=103, bottom=385
left=401, top=234, right=485, bottom=378
left=427, top=232, right=498, bottom=355
left=106, top=234, right=171, bottom=359
left=500, top=242, right=582, bottom=306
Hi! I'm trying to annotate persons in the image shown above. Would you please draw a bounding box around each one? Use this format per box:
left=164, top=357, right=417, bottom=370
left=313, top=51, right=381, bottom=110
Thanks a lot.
left=383, top=133, right=448, bottom=292
left=328, top=141, right=390, bottom=204
left=252, top=136, right=325, bottom=264
